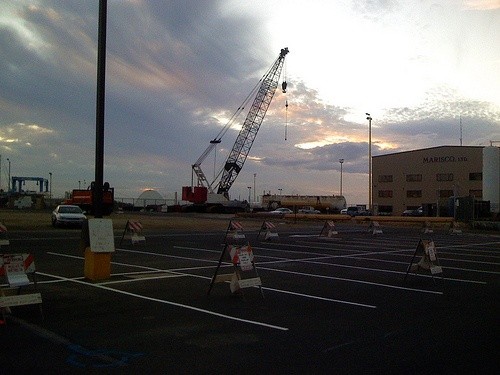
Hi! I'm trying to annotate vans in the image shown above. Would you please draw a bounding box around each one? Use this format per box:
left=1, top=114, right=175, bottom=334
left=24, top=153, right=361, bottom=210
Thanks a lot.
left=402, top=210, right=417, bottom=216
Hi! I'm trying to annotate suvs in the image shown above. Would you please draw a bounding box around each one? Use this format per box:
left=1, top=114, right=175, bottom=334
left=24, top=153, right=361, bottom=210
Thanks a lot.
left=418, top=207, right=423, bottom=216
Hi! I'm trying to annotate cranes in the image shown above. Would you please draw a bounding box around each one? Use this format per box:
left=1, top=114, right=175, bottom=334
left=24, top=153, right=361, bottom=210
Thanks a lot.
left=181, top=47, right=289, bottom=204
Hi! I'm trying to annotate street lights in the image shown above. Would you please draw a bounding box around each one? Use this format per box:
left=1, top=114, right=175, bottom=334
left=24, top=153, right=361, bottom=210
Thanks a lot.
left=247, top=186, right=251, bottom=205
left=49, top=173, right=52, bottom=207
left=6, top=158, right=10, bottom=192
left=366, top=112, right=373, bottom=208
left=278, top=189, right=282, bottom=195
left=338, top=158, right=344, bottom=196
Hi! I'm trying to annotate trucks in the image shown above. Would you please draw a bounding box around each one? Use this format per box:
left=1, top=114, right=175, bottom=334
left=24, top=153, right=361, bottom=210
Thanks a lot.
left=59, top=189, right=113, bottom=216
left=348, top=206, right=370, bottom=218
left=302, top=206, right=320, bottom=214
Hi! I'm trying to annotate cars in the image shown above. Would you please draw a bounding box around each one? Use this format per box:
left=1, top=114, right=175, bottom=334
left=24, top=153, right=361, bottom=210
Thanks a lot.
left=51, top=205, right=88, bottom=226
left=341, top=209, right=348, bottom=215
left=271, top=208, right=293, bottom=214
